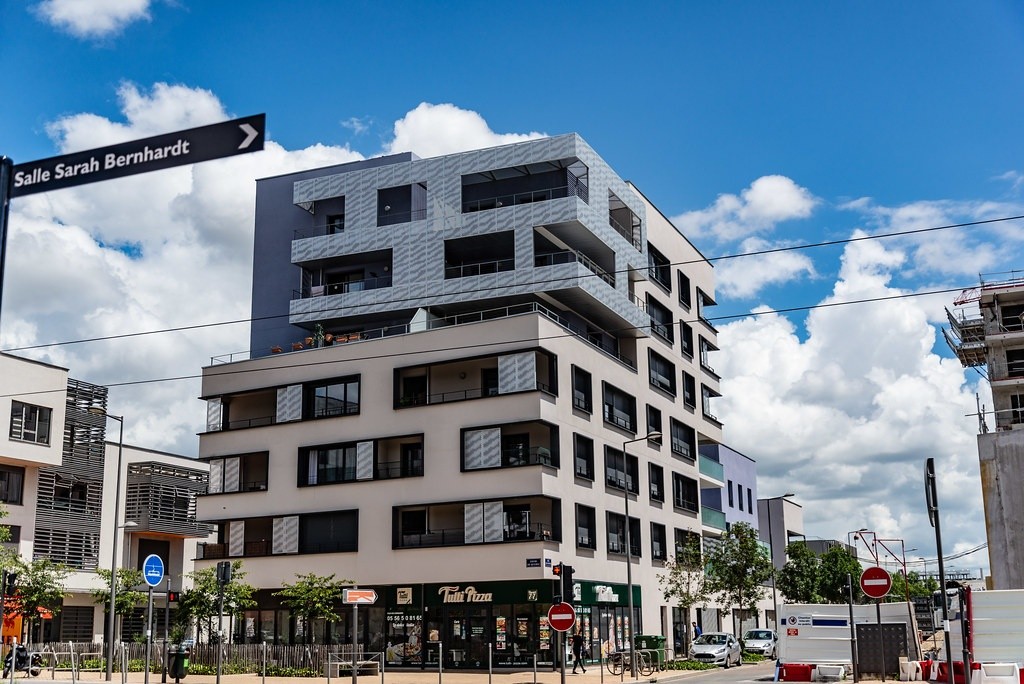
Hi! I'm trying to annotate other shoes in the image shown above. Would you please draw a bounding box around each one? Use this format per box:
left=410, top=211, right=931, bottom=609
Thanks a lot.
left=572, top=672, right=579, bottom=674
left=583, top=669, right=586, bottom=673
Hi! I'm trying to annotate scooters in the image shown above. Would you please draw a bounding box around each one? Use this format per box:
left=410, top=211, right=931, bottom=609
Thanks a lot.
left=3, top=641, right=42, bottom=679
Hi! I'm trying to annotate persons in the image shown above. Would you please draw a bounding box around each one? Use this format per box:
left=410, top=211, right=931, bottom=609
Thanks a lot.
left=571, top=629, right=587, bottom=674
left=692, top=622, right=702, bottom=640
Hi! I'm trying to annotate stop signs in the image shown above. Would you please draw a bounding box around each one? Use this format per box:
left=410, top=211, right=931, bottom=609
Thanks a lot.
left=859, top=566, right=892, bottom=599
left=548, top=602, right=576, bottom=631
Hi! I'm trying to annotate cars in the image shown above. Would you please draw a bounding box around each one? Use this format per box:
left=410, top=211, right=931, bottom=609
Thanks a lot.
left=689, top=632, right=742, bottom=669
left=743, top=628, right=778, bottom=661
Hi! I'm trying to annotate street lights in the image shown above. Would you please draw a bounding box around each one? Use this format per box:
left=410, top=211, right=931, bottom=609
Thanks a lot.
left=767, top=492, right=795, bottom=630
left=623, top=431, right=664, bottom=675
left=87, top=403, right=138, bottom=681
left=918, top=557, right=929, bottom=594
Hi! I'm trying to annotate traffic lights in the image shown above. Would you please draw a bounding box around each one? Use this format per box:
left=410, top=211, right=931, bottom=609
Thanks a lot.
left=552, top=565, right=562, bottom=576
left=168, top=591, right=179, bottom=602
left=562, top=565, right=575, bottom=603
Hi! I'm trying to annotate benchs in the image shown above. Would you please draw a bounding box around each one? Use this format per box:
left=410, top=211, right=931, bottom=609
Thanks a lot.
left=323, top=660, right=379, bottom=677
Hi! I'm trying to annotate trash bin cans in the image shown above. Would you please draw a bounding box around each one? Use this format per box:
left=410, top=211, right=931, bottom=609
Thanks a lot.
left=634, top=634, right=666, bottom=669
left=168, top=643, right=189, bottom=678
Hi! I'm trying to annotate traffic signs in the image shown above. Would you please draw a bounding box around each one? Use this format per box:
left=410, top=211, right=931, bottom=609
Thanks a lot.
left=8, top=112, right=267, bottom=200
left=347, top=589, right=379, bottom=603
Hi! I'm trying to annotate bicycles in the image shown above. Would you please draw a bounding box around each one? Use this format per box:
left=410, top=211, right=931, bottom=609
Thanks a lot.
left=606, top=644, right=655, bottom=677
